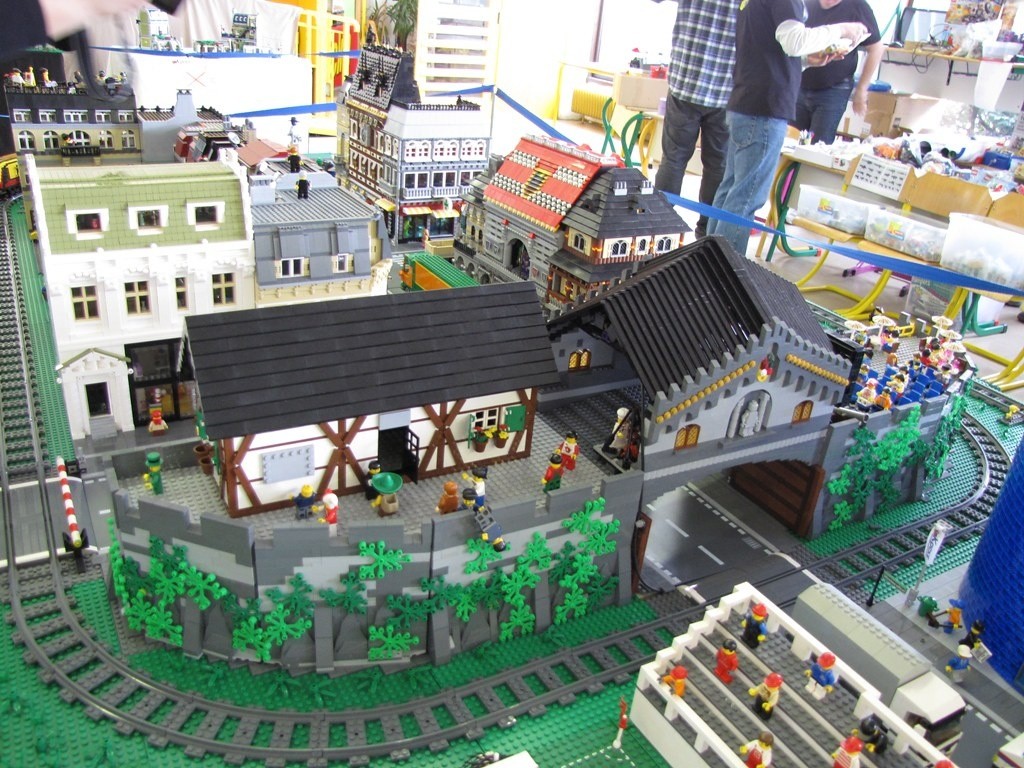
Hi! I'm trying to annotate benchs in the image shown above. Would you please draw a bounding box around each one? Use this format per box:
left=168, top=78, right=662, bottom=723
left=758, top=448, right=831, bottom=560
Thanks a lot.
left=572, top=80, right=656, bottom=142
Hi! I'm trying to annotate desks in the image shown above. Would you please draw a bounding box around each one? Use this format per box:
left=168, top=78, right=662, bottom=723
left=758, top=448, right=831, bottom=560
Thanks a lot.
left=105, top=42, right=313, bottom=146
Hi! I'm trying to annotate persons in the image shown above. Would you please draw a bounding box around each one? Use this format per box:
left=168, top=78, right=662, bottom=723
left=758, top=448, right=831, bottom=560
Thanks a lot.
left=794, top=0, right=883, bottom=146
left=0, top=1, right=145, bottom=153
left=706, top=1, right=869, bottom=257
left=655, top=0, right=737, bottom=241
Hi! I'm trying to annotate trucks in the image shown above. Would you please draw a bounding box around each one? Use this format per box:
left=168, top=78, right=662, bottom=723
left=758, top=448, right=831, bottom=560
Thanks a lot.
left=793, top=583, right=967, bottom=761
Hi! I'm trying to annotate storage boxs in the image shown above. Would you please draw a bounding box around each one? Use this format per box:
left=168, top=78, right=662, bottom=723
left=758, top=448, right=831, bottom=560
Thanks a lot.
left=607, top=71, right=709, bottom=178
left=773, top=85, right=1024, bottom=296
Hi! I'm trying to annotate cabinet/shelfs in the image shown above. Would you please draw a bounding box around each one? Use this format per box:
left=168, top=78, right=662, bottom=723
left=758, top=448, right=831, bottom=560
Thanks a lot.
left=849, top=0, right=1024, bottom=112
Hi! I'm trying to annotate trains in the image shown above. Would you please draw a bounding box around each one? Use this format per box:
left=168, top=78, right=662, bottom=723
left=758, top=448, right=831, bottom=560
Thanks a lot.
left=0, top=153, right=22, bottom=198
